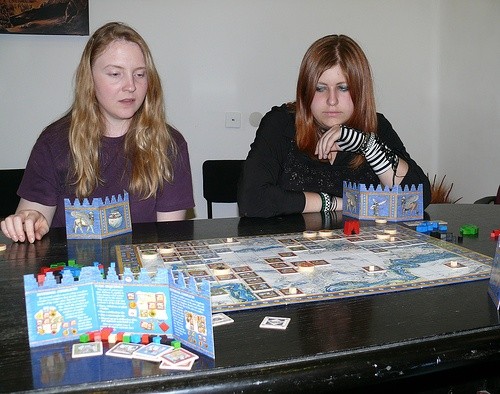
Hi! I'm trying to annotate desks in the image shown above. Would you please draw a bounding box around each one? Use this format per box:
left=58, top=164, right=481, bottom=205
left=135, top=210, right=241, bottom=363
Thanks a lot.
left=0, top=203, right=500, bottom=394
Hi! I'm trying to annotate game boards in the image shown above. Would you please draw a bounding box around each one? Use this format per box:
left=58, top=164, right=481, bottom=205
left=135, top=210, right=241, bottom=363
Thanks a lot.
left=114, top=223, right=495, bottom=314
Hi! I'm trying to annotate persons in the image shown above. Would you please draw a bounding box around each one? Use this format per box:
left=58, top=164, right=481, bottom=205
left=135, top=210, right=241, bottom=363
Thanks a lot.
left=4, top=220, right=195, bottom=259
left=1, top=22, right=195, bottom=244
left=237, top=34, right=431, bottom=217
left=238, top=210, right=431, bottom=357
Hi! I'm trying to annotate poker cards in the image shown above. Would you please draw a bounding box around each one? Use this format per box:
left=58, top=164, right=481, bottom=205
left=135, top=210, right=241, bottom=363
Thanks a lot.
left=72, top=341, right=103, bottom=358
left=259, top=316, right=290, bottom=330
left=105, top=342, right=145, bottom=359
left=212, top=313, right=234, bottom=328
left=132, top=342, right=199, bottom=370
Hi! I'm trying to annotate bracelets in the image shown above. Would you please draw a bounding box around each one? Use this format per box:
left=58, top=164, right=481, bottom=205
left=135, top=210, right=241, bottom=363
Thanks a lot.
left=320, top=210, right=338, bottom=230
left=319, top=192, right=337, bottom=212
left=356, top=131, right=375, bottom=156
